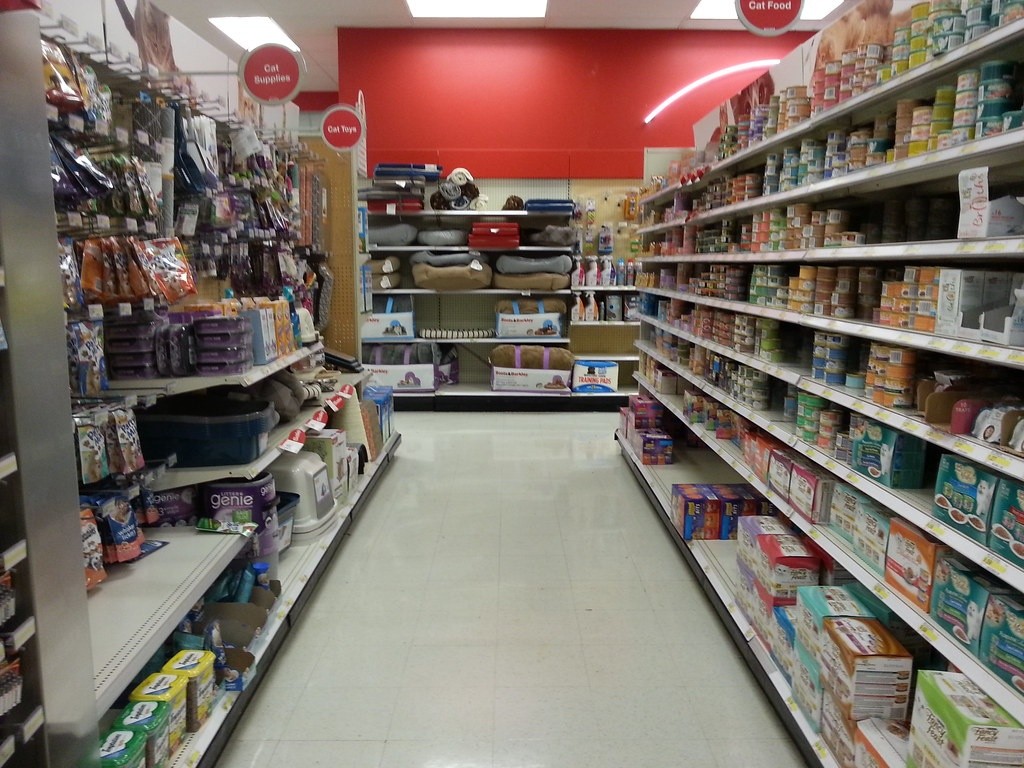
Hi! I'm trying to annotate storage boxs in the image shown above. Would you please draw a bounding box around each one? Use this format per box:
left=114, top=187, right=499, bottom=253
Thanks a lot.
left=361, top=363, right=439, bottom=392
left=277, top=487, right=301, bottom=555
left=80, top=729, right=148, bottom=768
left=604, top=294, right=623, bottom=321
left=364, top=385, right=396, bottom=437
left=237, top=300, right=293, bottom=366
left=129, top=673, right=190, bottom=759
left=363, top=395, right=387, bottom=444
left=495, top=311, right=562, bottom=338
left=111, top=700, right=171, bottom=768
left=624, top=294, right=640, bottom=322
left=619, top=356, right=694, bottom=465
left=160, top=649, right=216, bottom=733
left=573, top=361, right=619, bottom=393
left=671, top=391, right=1024, bottom=768
left=364, top=266, right=373, bottom=311
left=361, top=310, right=417, bottom=339
left=302, top=429, right=348, bottom=511
left=490, top=366, right=572, bottom=395
left=957, top=166, right=1024, bottom=239
left=345, top=445, right=359, bottom=494
left=439, top=357, right=459, bottom=385
left=357, top=207, right=369, bottom=253
left=360, top=265, right=365, bottom=313
left=937, top=268, right=1023, bottom=322
left=364, top=389, right=390, bottom=440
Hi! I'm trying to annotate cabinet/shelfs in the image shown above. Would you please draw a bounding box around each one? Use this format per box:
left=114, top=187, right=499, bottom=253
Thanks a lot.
left=613, top=17, right=1024, bottom=768
left=0, top=369, right=402, bottom=768
left=366, top=207, right=641, bottom=409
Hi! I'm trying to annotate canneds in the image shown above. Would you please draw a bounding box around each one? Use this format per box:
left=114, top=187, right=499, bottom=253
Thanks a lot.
left=659, top=0, right=1024, bottom=452
